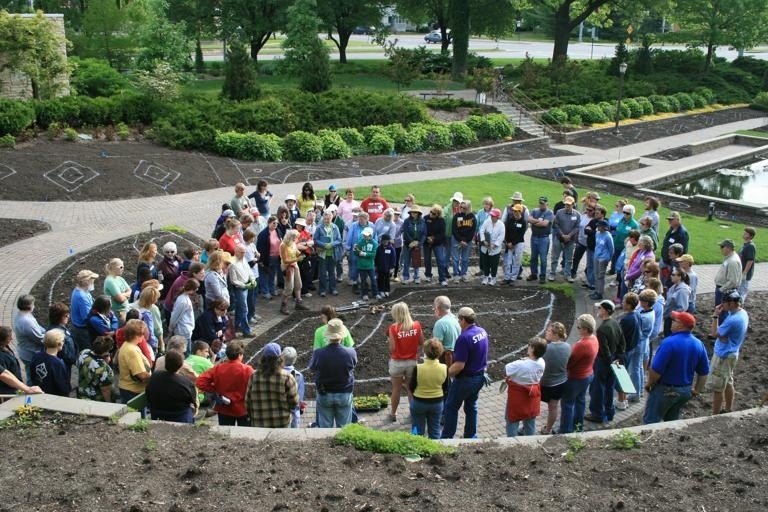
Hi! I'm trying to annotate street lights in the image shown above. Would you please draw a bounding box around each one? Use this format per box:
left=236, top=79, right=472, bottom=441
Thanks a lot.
left=610, top=59, right=628, bottom=136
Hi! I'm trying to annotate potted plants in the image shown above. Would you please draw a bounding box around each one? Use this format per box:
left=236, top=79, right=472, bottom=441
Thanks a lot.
left=352, top=394, right=389, bottom=412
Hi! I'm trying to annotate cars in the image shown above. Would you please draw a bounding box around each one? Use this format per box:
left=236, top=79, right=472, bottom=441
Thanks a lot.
left=352, top=25, right=375, bottom=34
left=422, top=31, right=452, bottom=44
left=430, top=27, right=451, bottom=33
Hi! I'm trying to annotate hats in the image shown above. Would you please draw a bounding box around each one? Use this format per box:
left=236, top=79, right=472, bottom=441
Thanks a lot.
left=669, top=307, right=700, bottom=326
left=719, top=237, right=736, bottom=249
left=77, top=269, right=99, bottom=282
left=595, top=298, right=614, bottom=316
left=595, top=218, right=608, bottom=226
left=721, top=286, right=739, bottom=302
left=285, top=183, right=421, bottom=238
left=675, top=254, right=696, bottom=264
left=447, top=191, right=465, bottom=204
left=321, top=318, right=351, bottom=340
left=537, top=191, right=605, bottom=206
left=666, top=211, right=681, bottom=221
left=490, top=190, right=525, bottom=219
left=141, top=278, right=164, bottom=291
left=220, top=206, right=261, bottom=215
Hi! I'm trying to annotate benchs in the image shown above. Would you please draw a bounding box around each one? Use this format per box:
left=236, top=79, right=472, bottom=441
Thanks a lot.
left=419, top=93, right=454, bottom=100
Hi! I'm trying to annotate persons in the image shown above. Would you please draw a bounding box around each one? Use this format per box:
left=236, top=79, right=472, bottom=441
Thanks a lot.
left=1, top=176, right=756, bottom=440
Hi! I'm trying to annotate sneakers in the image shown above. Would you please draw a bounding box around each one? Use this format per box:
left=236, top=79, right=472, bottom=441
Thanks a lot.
left=612, top=397, right=631, bottom=413
left=249, top=313, right=262, bottom=324
left=306, top=284, right=392, bottom=303
left=262, top=289, right=279, bottom=300
left=280, top=300, right=311, bottom=314
left=585, top=413, right=604, bottom=424
left=244, top=332, right=256, bottom=338
left=402, top=270, right=596, bottom=291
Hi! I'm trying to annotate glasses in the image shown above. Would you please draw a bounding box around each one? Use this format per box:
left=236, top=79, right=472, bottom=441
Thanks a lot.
left=209, top=247, right=218, bottom=249
left=218, top=307, right=228, bottom=309
left=430, top=211, right=438, bottom=215
left=165, top=251, right=178, bottom=254
left=114, top=266, right=123, bottom=268
left=459, top=206, right=466, bottom=208
